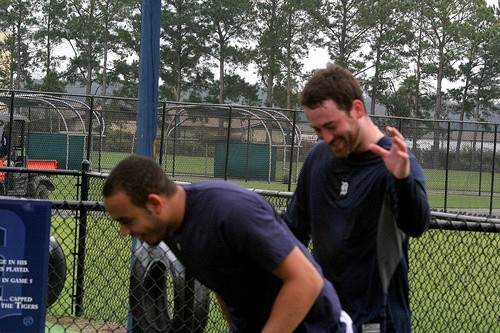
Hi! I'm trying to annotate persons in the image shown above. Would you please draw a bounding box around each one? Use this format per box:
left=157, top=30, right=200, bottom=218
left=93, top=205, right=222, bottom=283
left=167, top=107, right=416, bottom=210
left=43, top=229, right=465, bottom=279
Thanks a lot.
left=283, top=64, right=430, bottom=333
left=101, top=155, right=357, bottom=333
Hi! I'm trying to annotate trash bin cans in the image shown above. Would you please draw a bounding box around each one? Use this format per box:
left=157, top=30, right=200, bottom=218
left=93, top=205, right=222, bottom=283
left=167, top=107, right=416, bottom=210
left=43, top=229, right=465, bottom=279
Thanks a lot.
left=0, top=199, right=52, bottom=333
